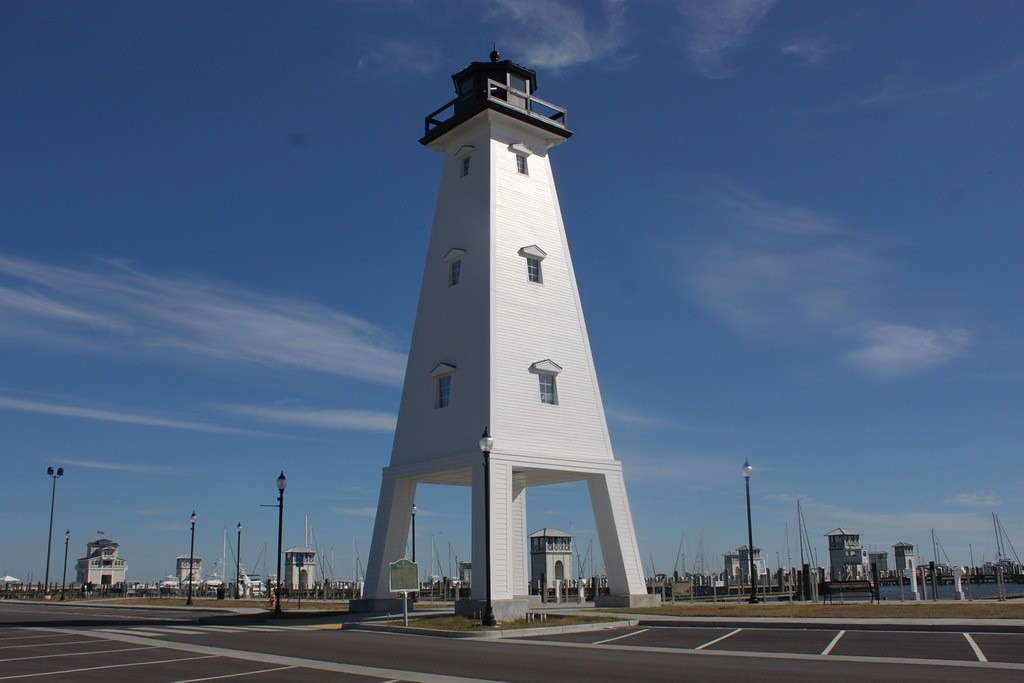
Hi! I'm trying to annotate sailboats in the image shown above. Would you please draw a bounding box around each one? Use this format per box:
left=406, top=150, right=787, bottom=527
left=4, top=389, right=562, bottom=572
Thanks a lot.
left=930, top=512, right=1024, bottom=574
left=206, top=528, right=278, bottom=597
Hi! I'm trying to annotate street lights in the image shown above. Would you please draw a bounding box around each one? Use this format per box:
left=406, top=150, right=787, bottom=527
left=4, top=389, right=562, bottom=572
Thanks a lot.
left=741, top=458, right=759, bottom=603
left=186, top=510, right=197, bottom=606
left=411, top=504, right=417, bottom=561
left=235, top=522, right=242, bottom=599
left=60, top=529, right=71, bottom=600
left=274, top=470, right=287, bottom=618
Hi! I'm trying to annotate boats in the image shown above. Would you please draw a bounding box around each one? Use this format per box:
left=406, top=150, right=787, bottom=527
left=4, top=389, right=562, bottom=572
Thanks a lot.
left=159, top=575, right=180, bottom=587
